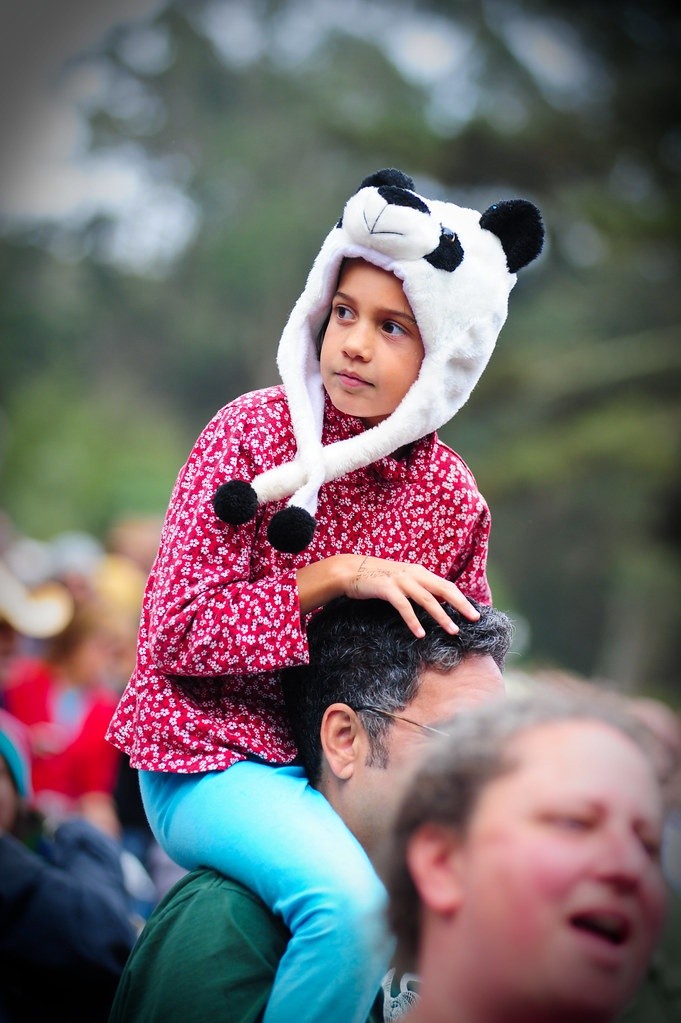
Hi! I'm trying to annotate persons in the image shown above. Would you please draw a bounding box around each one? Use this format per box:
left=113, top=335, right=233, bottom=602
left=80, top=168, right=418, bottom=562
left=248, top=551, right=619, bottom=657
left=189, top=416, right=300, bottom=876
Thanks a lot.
left=0, top=523, right=182, bottom=1023
left=114, top=586, right=514, bottom=1023
left=101, top=168, right=545, bottom=1022
left=388, top=668, right=681, bottom=1023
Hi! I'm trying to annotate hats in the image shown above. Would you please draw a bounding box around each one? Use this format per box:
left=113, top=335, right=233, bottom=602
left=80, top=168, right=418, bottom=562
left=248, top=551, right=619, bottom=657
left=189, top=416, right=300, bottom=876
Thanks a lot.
left=213, top=168, right=544, bottom=553
